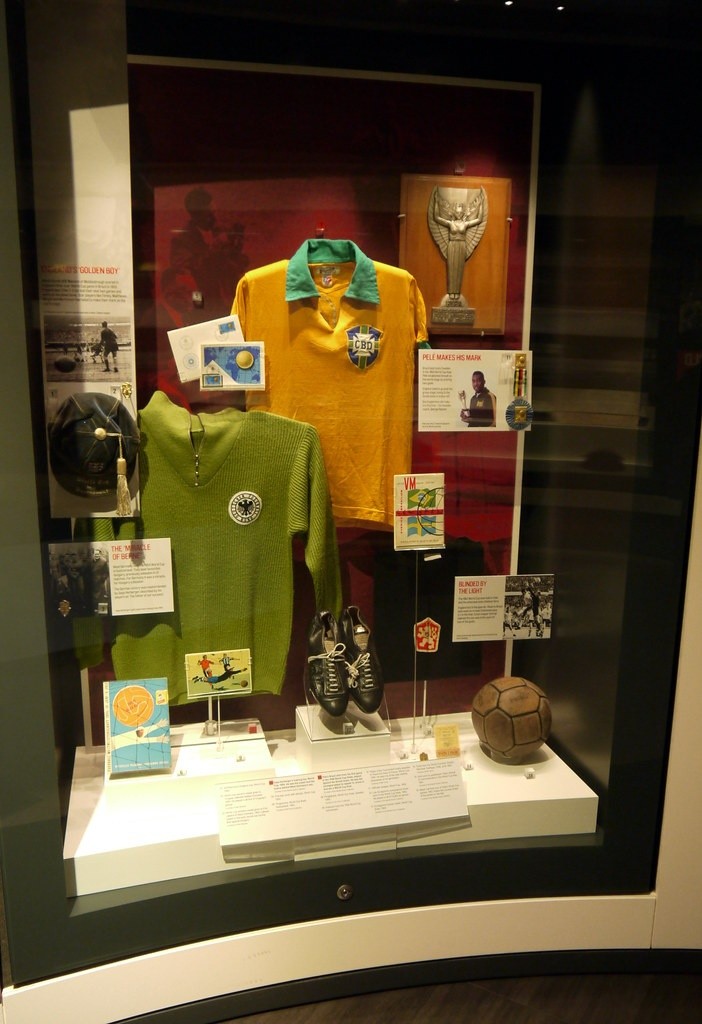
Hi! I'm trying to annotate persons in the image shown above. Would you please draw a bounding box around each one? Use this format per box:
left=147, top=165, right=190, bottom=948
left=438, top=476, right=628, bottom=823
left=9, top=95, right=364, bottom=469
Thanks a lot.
left=192, top=654, right=247, bottom=688
left=434, top=193, right=483, bottom=299
left=503, top=576, right=552, bottom=639
left=49, top=547, right=109, bottom=610
left=163, top=187, right=252, bottom=327
left=459, top=370, right=497, bottom=428
left=61, top=319, right=119, bottom=373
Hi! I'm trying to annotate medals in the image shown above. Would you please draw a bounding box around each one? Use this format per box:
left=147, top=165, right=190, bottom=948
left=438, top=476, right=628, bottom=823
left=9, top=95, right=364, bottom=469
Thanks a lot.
left=506, top=400, right=532, bottom=430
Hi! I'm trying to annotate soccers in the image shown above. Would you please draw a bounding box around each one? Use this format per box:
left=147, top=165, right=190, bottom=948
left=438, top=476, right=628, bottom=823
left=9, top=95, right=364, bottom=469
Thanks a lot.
left=469, top=676, right=554, bottom=760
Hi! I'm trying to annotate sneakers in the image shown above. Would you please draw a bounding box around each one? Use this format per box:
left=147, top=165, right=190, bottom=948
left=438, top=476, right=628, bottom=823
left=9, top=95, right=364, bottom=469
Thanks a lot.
left=340, top=606, right=383, bottom=714
left=307, top=611, right=348, bottom=717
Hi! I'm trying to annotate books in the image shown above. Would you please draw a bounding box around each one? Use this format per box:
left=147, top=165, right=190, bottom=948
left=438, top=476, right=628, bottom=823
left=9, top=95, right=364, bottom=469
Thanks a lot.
left=102, top=677, right=173, bottom=779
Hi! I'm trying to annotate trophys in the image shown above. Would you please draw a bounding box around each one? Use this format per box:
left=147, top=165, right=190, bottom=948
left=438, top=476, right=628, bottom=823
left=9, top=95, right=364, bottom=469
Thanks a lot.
left=458, top=390, right=470, bottom=418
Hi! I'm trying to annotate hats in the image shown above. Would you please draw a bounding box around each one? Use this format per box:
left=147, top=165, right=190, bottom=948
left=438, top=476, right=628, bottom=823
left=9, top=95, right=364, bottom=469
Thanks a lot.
left=48, top=390, right=140, bottom=515
